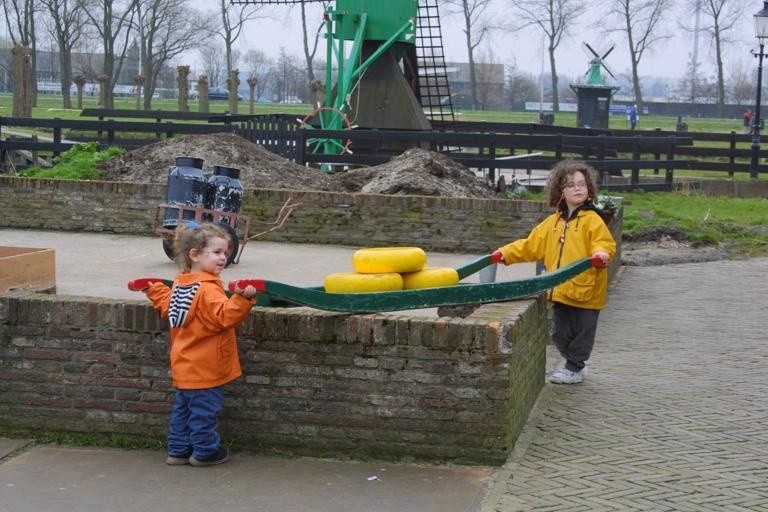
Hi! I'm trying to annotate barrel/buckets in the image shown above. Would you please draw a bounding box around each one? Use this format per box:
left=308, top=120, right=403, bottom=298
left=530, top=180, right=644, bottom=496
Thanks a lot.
left=207, top=164, right=245, bottom=233
left=164, top=156, right=208, bottom=226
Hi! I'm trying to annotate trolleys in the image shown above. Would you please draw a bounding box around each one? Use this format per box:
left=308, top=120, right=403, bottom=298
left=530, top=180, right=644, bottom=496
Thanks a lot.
left=150, top=195, right=303, bottom=266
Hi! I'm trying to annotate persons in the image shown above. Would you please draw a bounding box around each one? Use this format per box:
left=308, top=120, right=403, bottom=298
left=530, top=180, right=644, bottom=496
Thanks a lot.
left=744, top=109, right=752, bottom=135
left=142, top=222, right=258, bottom=466
left=630, top=104, right=638, bottom=130
left=492, top=158, right=618, bottom=386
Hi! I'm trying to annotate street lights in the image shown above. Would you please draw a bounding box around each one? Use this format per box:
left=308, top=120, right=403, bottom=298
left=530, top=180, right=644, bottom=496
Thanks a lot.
left=748, top=1, right=767, bottom=178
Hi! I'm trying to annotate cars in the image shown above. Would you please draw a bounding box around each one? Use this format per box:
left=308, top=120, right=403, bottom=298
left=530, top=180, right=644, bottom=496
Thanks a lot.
left=193, top=88, right=243, bottom=101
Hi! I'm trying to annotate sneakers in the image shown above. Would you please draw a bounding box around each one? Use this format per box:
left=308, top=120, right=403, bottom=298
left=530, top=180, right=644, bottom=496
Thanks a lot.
left=548, top=364, right=589, bottom=384
left=166, top=445, right=230, bottom=467
